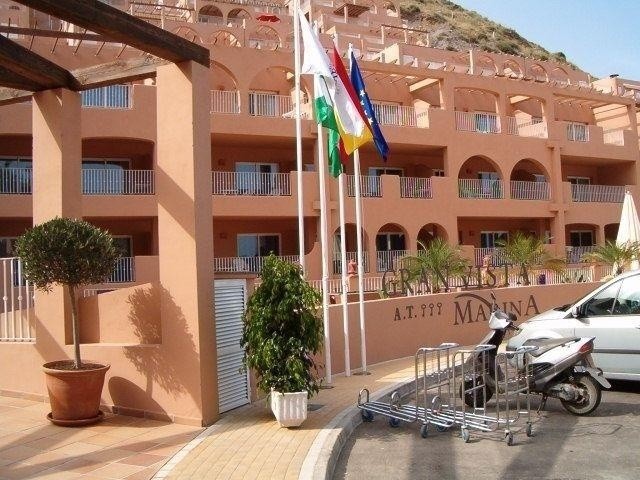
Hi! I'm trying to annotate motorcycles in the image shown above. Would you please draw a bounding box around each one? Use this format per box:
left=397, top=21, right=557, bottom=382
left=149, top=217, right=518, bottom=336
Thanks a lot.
left=459, top=290, right=612, bottom=417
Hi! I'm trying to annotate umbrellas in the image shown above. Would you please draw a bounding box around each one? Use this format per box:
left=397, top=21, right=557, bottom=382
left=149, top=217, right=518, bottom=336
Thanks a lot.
left=612, top=191, right=640, bottom=272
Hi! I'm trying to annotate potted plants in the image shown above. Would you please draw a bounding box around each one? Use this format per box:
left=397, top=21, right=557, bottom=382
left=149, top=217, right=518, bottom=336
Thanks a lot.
left=13, top=216, right=127, bottom=428
left=240, top=252, right=325, bottom=427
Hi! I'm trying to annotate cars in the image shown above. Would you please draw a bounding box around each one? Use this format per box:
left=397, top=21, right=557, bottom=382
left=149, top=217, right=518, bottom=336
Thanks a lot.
left=506, top=269, right=640, bottom=383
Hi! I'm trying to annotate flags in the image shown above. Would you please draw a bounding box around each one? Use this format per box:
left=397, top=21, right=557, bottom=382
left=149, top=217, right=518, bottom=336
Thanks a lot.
left=349, top=50, right=390, bottom=165
left=332, top=43, right=374, bottom=167
left=299, top=8, right=374, bottom=156
left=313, top=74, right=344, bottom=178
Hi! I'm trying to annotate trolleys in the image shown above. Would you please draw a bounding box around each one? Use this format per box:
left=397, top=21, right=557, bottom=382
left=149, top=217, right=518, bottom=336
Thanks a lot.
left=357, top=342, right=539, bottom=445
